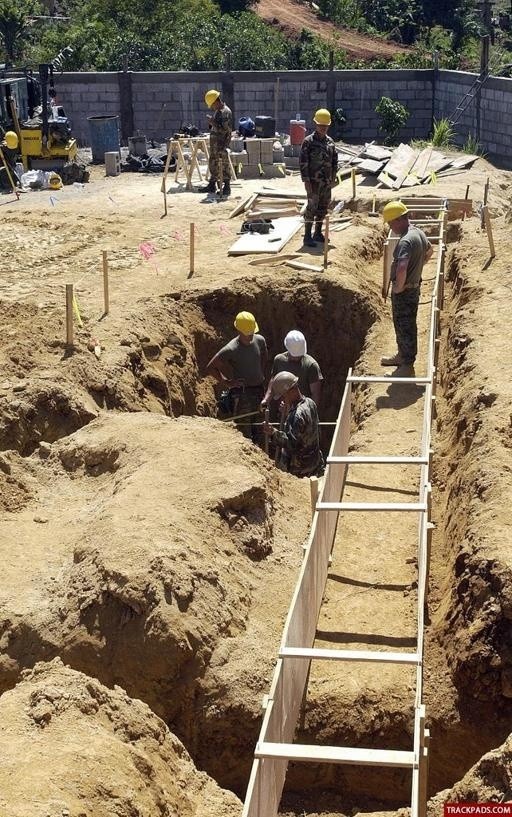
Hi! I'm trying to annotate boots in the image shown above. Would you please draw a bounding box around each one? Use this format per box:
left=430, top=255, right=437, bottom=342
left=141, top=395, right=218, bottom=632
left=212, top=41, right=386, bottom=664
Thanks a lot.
left=303, top=222, right=317, bottom=247
left=217, top=180, right=231, bottom=195
left=197, top=182, right=218, bottom=193
left=313, top=221, right=330, bottom=243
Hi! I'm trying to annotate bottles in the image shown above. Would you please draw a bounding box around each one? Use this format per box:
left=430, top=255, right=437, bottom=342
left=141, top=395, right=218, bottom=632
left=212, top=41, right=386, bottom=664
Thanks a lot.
left=333, top=200, right=344, bottom=213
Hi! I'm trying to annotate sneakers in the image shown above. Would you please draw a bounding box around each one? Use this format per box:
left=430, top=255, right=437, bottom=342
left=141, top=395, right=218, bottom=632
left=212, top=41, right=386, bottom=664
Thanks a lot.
left=383, top=363, right=416, bottom=378
left=381, top=351, right=415, bottom=367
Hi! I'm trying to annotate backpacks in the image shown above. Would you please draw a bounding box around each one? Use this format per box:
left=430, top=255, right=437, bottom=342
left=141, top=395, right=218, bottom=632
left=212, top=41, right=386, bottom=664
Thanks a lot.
left=238, top=116, right=257, bottom=138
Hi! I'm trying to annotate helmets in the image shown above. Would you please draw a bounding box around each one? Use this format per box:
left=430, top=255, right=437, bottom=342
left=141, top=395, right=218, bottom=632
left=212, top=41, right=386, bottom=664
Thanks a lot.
left=382, top=200, right=409, bottom=225
left=283, top=329, right=308, bottom=358
left=270, top=370, right=300, bottom=400
left=312, top=108, right=332, bottom=126
left=233, top=310, right=260, bottom=336
left=4, top=131, right=19, bottom=149
left=47, top=174, right=64, bottom=189
left=204, top=89, right=223, bottom=108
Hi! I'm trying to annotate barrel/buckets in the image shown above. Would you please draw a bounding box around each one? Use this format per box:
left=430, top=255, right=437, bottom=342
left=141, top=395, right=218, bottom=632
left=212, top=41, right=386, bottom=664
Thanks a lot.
left=289, top=119, right=306, bottom=144
left=239, top=115, right=256, bottom=137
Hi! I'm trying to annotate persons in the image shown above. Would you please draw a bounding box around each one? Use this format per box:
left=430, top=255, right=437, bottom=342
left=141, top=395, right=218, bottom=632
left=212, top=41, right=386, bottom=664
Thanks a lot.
left=271, top=371, right=325, bottom=478
left=204, top=311, right=267, bottom=452
left=198, top=90, right=233, bottom=195
left=261, top=330, right=325, bottom=460
left=299, top=109, right=337, bottom=248
left=382, top=201, right=433, bottom=366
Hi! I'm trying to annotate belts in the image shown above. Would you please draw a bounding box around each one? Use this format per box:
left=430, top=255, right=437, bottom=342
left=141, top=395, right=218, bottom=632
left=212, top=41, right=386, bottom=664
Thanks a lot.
left=229, top=384, right=260, bottom=391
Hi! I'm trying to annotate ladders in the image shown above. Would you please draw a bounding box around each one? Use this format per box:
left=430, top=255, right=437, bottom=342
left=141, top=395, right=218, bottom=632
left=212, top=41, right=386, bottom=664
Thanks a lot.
left=446, top=46, right=508, bottom=128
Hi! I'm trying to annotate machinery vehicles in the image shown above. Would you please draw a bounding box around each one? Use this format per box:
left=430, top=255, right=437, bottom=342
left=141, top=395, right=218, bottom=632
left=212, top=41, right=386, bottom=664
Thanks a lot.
left=0, top=62, right=94, bottom=189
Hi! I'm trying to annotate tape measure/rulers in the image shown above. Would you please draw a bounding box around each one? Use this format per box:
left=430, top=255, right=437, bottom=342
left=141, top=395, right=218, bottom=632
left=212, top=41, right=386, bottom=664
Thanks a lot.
left=222, top=410, right=259, bottom=422
